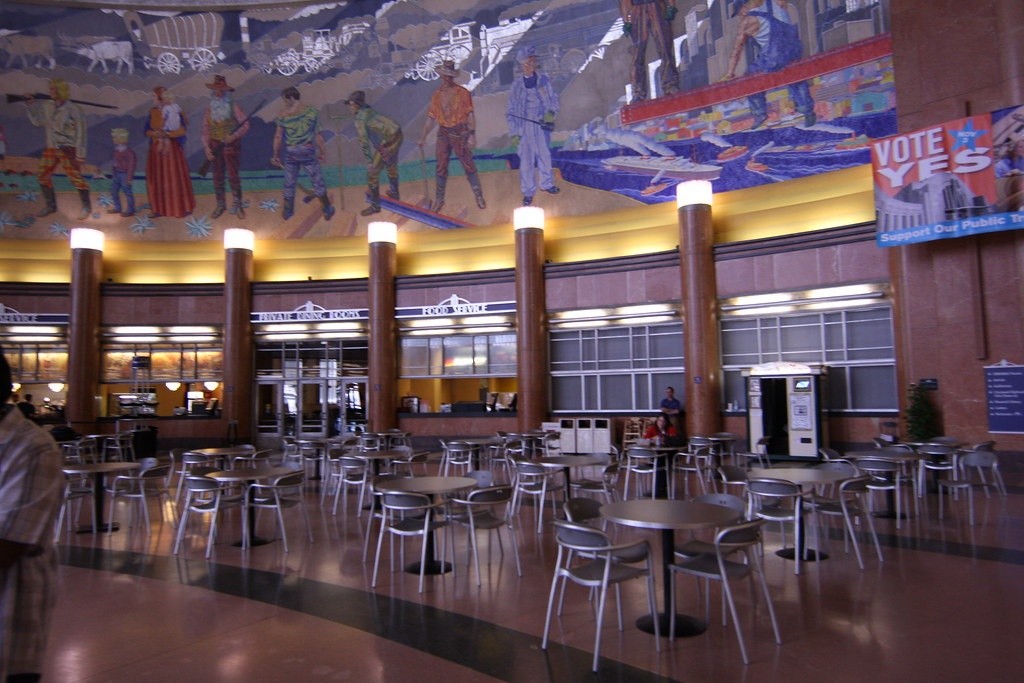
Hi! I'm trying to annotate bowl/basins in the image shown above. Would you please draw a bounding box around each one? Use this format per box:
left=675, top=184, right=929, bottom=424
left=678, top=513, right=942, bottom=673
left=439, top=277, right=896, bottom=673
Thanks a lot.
left=636, top=439, right=652, bottom=445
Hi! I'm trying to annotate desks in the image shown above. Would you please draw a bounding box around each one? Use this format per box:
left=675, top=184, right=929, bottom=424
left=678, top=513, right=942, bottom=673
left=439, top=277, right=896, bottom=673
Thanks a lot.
left=598, top=500, right=740, bottom=638
left=191, top=447, right=254, bottom=495
left=204, top=467, right=292, bottom=547
left=292, top=438, right=342, bottom=480
left=361, top=432, right=402, bottom=465
left=79, top=434, right=126, bottom=477
left=62, top=462, right=141, bottom=533
left=375, top=477, right=478, bottom=576
left=844, top=452, right=931, bottom=519
left=342, top=451, right=409, bottom=510
left=448, top=438, right=506, bottom=470
left=518, top=456, right=605, bottom=521
left=746, top=469, right=851, bottom=561
left=898, top=441, right=970, bottom=494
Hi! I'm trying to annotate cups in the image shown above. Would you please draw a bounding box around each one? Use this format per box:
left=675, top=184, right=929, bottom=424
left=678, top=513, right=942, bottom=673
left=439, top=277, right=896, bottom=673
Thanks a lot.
left=656, top=438, right=660, bottom=445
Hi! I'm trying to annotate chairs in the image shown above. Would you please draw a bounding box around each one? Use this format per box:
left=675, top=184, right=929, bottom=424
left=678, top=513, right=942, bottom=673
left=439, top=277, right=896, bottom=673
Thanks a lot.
left=54, top=419, right=1006, bottom=672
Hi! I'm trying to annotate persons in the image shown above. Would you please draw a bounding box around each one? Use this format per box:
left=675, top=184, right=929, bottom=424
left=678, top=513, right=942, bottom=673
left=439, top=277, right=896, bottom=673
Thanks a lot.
left=642, top=413, right=678, bottom=498
left=0, top=345, right=65, bottom=683
left=660, top=387, right=681, bottom=427
left=17, top=394, right=34, bottom=418
left=7, top=394, right=19, bottom=404
